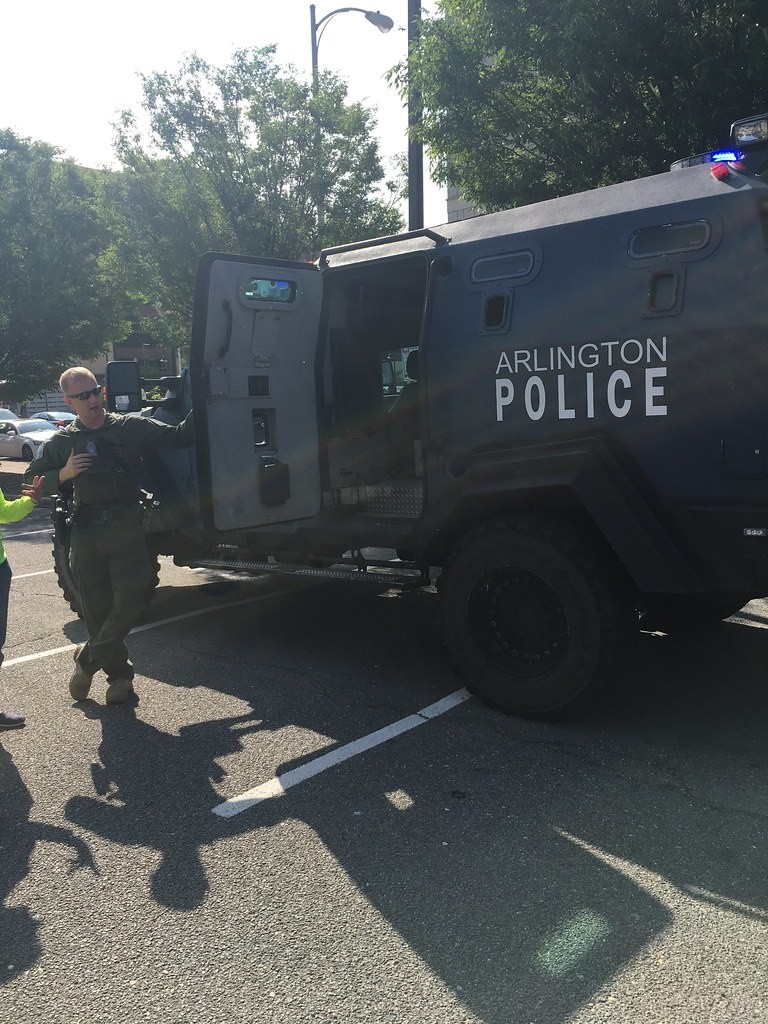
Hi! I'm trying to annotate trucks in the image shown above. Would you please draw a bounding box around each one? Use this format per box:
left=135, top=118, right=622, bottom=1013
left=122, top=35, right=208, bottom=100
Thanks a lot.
left=49, top=100, right=767, bottom=722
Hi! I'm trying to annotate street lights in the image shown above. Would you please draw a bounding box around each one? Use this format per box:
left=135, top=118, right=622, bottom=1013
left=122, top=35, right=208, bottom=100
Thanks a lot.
left=309, top=5, right=396, bottom=245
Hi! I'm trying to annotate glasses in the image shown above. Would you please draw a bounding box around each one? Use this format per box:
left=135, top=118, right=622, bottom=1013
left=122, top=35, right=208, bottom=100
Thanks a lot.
left=67, top=385, right=101, bottom=400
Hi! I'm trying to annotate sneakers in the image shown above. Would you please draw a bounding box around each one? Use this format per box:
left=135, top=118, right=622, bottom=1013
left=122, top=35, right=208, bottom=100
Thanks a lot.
left=105, top=677, right=133, bottom=702
left=69, top=648, right=92, bottom=701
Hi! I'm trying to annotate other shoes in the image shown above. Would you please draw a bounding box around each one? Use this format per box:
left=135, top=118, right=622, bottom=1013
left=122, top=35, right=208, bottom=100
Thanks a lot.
left=0, top=713, right=25, bottom=726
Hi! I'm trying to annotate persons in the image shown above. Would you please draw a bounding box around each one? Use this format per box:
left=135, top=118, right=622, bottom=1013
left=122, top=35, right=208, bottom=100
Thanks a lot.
left=23, top=366, right=199, bottom=705
left=0, top=475, right=48, bottom=728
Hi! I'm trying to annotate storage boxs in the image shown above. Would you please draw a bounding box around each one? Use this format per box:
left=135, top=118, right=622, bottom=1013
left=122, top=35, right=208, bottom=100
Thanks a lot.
left=319, top=426, right=390, bottom=489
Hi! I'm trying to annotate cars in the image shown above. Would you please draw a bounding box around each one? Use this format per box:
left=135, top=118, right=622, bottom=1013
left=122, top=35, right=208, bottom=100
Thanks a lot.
left=29, top=411, right=78, bottom=429
left=0, top=418, right=61, bottom=460
left=0, top=408, right=22, bottom=421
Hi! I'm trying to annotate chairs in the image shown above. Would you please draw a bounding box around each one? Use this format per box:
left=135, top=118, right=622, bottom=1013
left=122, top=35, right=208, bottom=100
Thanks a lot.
left=388, top=349, right=423, bottom=427
